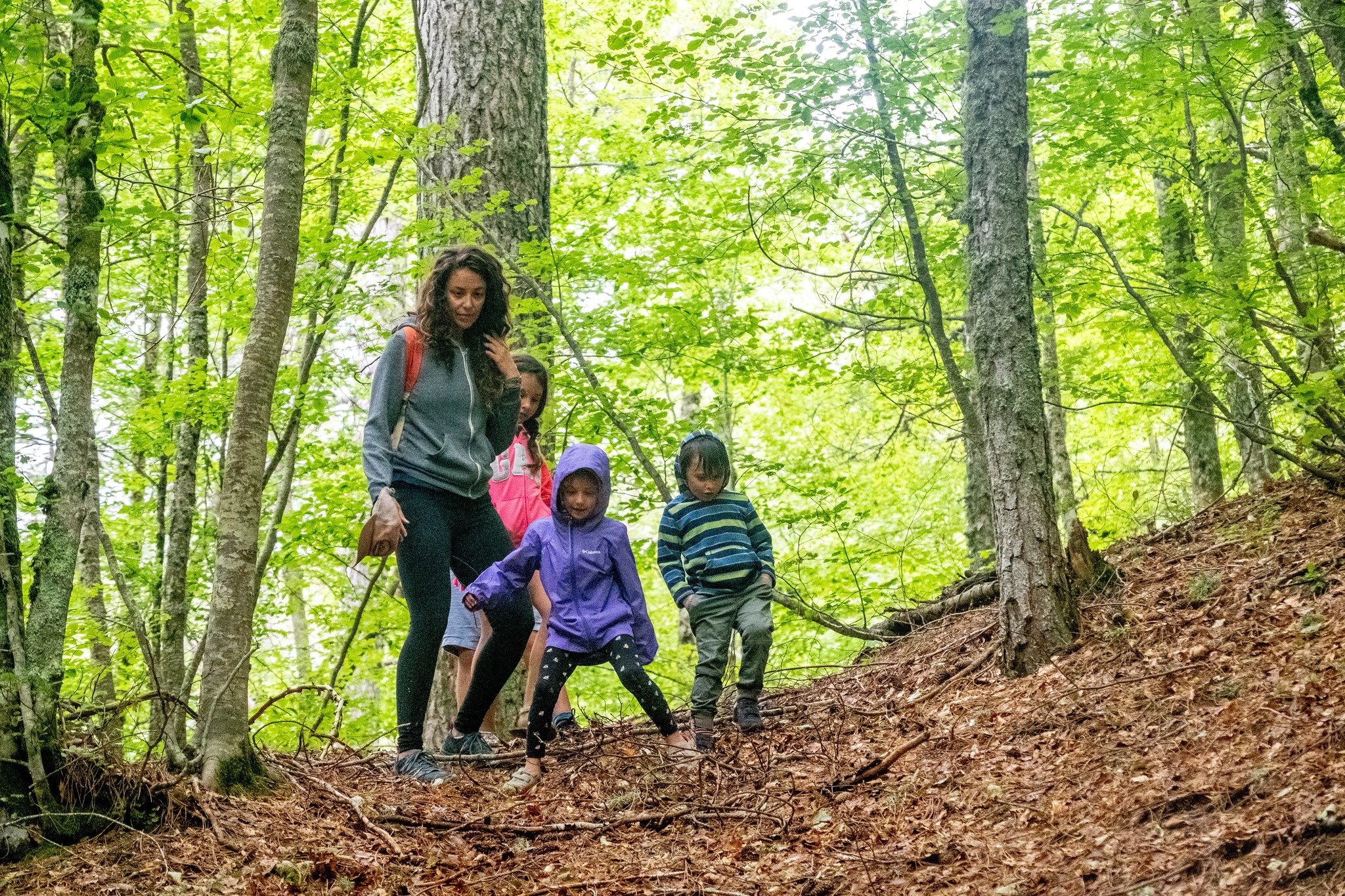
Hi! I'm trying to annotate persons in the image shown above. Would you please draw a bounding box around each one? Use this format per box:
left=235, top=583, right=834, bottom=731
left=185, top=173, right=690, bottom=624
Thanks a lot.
left=462, top=442, right=697, bottom=795
left=439, top=353, right=583, bottom=757
left=359, top=245, right=537, bottom=788
left=657, top=428, right=777, bottom=755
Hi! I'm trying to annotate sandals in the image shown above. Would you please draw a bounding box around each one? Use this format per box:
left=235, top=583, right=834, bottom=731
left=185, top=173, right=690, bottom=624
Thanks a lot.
left=507, top=707, right=557, bottom=740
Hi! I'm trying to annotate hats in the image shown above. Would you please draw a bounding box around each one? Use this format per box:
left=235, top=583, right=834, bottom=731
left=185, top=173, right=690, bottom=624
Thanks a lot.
left=350, top=484, right=403, bottom=569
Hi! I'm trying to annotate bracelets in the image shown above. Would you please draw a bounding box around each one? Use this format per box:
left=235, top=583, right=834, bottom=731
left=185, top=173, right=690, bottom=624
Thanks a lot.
left=502, top=376, right=523, bottom=388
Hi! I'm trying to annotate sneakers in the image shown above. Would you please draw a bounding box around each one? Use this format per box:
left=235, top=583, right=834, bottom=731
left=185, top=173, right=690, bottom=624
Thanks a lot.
left=693, top=725, right=715, bottom=751
left=732, top=698, right=764, bottom=732
left=668, top=735, right=698, bottom=773
left=553, top=708, right=582, bottom=741
left=440, top=729, right=501, bottom=767
left=502, top=766, right=543, bottom=795
left=393, top=748, right=457, bottom=786
